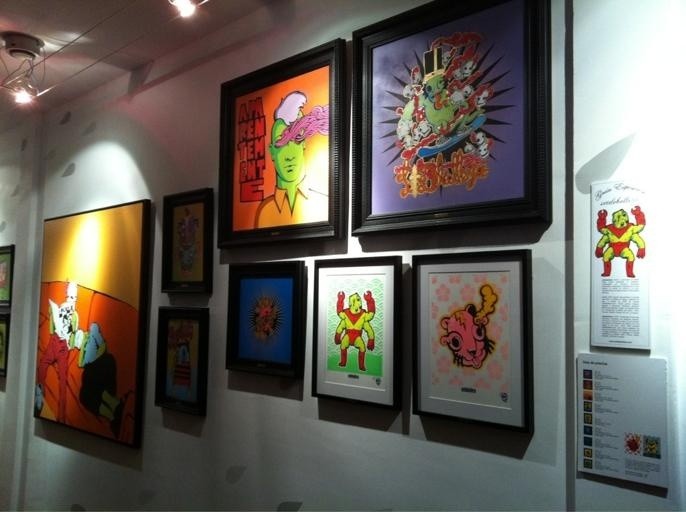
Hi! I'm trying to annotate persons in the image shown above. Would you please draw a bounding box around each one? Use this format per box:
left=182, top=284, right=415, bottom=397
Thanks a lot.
left=72, top=321, right=126, bottom=439
left=254, top=87, right=331, bottom=230
left=36, top=281, right=80, bottom=424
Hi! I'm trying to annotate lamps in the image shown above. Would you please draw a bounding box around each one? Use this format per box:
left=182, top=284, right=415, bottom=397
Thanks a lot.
left=2, top=31, right=43, bottom=104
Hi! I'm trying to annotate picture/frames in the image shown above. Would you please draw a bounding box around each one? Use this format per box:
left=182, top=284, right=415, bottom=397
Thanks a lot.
left=161, top=183, right=215, bottom=297
left=350, top=0, right=551, bottom=237
left=217, top=35, right=348, bottom=247
left=311, top=256, right=407, bottom=414
left=412, top=249, right=537, bottom=436
left=224, top=259, right=307, bottom=387
left=31, top=199, right=155, bottom=446
left=0, top=311, right=12, bottom=386
left=152, top=306, right=205, bottom=419
left=0, top=244, right=17, bottom=304
